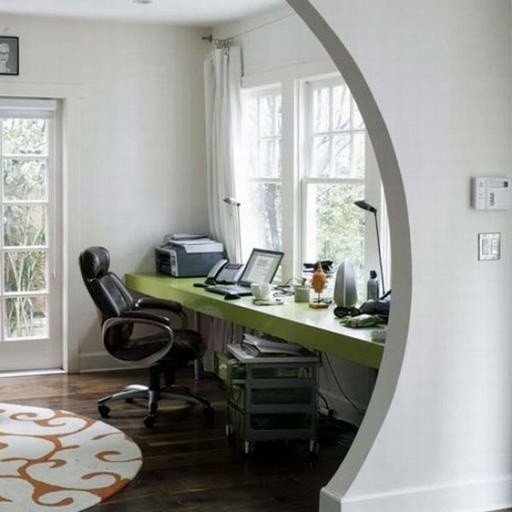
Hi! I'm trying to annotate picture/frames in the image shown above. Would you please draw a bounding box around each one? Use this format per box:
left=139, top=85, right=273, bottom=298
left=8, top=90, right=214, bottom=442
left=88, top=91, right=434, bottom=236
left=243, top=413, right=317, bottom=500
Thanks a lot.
left=0, top=34, right=20, bottom=75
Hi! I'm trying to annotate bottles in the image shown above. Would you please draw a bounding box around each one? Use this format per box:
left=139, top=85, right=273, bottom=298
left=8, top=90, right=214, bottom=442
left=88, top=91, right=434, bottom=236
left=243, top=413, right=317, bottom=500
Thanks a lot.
left=367, top=270, right=380, bottom=300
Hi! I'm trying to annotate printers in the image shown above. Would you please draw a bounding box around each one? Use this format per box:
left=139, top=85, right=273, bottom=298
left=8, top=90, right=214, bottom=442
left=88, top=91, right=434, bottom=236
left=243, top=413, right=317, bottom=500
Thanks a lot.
left=152, top=233, right=224, bottom=277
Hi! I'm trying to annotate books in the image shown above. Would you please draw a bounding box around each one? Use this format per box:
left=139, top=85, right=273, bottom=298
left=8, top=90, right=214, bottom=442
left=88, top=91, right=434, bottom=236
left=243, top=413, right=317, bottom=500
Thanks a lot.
left=240, top=333, right=303, bottom=357
left=169, top=234, right=216, bottom=245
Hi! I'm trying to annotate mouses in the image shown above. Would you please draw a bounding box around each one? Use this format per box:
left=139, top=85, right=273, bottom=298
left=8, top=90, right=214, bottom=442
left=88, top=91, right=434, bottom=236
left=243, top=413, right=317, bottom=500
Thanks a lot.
left=224, top=293, right=242, bottom=299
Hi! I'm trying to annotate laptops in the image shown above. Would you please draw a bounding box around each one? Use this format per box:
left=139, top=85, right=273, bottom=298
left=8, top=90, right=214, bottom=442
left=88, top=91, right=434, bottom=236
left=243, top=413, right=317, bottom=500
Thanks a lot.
left=206, top=248, right=284, bottom=294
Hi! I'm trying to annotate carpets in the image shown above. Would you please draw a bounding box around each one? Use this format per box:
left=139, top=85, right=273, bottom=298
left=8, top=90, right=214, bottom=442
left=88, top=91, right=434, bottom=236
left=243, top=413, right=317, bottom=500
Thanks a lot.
left=0, top=403, right=145, bottom=512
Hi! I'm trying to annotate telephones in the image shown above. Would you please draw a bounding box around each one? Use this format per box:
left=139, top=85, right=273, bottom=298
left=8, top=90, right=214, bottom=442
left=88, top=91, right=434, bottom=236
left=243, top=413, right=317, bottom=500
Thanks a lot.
left=206, top=259, right=246, bottom=285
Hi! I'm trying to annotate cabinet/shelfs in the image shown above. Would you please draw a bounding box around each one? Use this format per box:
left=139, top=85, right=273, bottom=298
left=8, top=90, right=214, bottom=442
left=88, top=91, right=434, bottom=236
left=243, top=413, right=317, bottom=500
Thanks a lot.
left=215, top=340, right=324, bottom=444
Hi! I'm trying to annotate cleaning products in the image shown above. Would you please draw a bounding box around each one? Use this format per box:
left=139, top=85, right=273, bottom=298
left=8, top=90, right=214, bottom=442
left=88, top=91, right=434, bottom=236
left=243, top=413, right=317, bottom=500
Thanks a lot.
left=366, top=270, right=378, bottom=299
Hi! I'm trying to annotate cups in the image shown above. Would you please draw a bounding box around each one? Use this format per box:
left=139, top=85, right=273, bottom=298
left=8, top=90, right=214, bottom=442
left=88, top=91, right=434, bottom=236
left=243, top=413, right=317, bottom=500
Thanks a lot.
left=292, top=283, right=310, bottom=303
left=250, top=281, right=271, bottom=299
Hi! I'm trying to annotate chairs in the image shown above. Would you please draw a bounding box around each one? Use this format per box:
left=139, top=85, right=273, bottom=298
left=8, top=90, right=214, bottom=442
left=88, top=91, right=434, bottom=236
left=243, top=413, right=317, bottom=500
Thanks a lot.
left=78, top=245, right=216, bottom=429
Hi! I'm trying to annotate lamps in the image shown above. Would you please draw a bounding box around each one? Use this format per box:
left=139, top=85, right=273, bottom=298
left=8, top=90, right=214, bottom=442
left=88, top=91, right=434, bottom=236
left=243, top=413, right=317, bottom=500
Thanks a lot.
left=353, top=199, right=389, bottom=301
left=222, top=195, right=247, bottom=268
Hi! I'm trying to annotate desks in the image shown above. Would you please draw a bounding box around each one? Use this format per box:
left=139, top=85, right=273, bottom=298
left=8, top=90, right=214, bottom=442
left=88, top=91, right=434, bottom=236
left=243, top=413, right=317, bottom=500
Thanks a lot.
left=122, top=272, right=384, bottom=371
